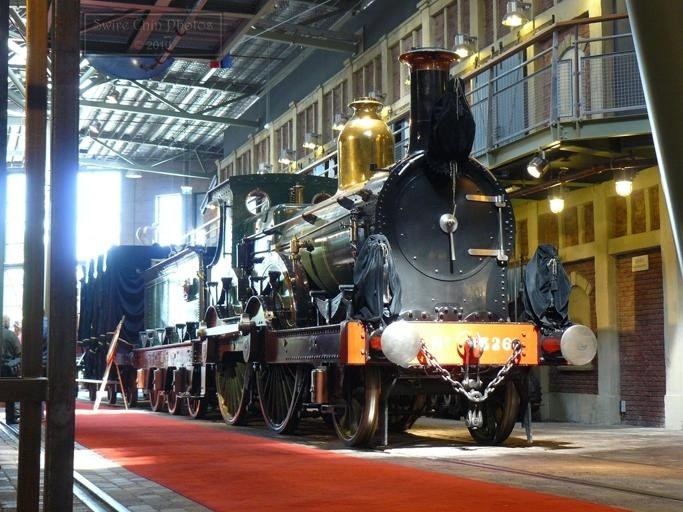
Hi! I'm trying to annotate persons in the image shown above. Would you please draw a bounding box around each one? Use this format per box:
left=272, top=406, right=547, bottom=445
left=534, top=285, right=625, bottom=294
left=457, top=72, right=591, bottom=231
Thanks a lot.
left=0, top=306, right=79, bottom=425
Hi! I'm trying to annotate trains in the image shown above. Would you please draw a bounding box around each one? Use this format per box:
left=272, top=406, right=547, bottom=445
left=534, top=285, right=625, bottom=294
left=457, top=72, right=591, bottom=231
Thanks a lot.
left=78, top=46, right=596, bottom=450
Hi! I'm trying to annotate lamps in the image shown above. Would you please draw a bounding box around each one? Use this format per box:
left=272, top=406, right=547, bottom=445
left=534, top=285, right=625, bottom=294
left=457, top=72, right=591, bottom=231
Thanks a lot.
left=451, top=33, right=477, bottom=57
left=500, top=1, right=532, bottom=27
left=277, top=113, right=346, bottom=166
left=527, top=149, right=551, bottom=181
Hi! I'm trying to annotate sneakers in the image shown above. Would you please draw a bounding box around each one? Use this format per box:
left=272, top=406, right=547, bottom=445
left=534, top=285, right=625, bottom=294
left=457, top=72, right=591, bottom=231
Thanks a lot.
left=6, top=417, right=21, bottom=425
